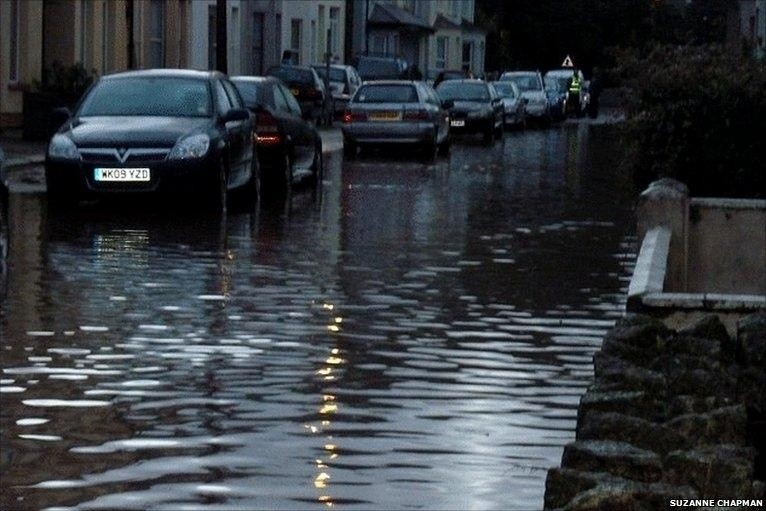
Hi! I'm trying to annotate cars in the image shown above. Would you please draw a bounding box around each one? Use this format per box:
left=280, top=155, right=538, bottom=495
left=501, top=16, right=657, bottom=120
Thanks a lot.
left=2, top=57, right=593, bottom=305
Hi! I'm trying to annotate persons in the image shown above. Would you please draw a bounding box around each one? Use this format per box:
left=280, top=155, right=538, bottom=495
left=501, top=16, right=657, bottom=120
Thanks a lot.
left=398, top=67, right=411, bottom=80
left=565, top=66, right=585, bottom=119
left=281, top=50, right=293, bottom=66
left=587, top=73, right=603, bottom=120
left=408, top=63, right=422, bottom=81
